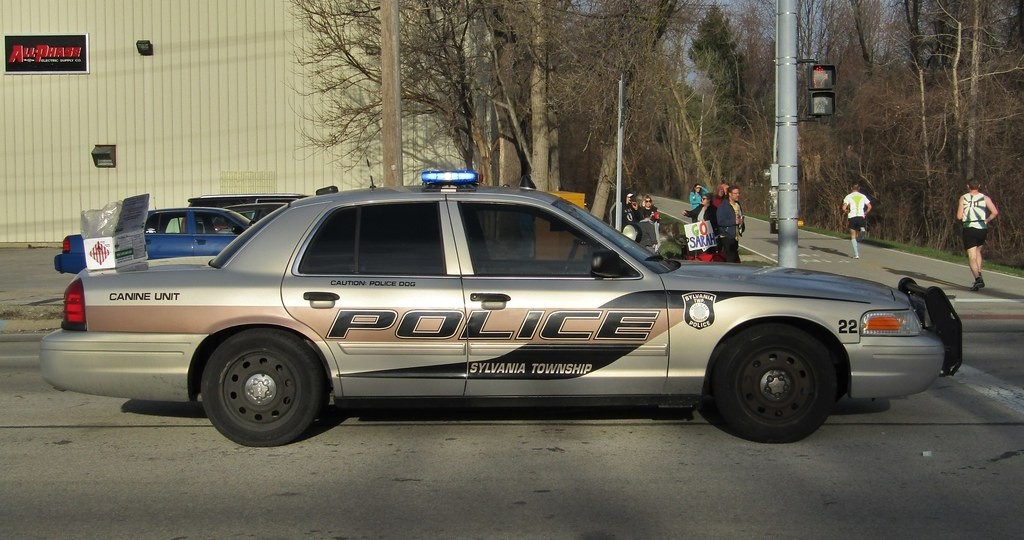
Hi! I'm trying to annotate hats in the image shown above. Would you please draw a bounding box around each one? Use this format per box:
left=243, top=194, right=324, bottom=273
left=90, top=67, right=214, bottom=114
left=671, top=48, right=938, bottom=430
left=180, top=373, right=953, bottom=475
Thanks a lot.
left=627, top=193, right=633, bottom=198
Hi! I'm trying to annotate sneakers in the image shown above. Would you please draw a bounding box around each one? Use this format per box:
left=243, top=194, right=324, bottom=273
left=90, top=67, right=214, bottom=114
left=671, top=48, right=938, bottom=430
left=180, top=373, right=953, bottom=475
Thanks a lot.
left=859, top=227, right=865, bottom=239
left=851, top=255, right=859, bottom=259
left=968, top=272, right=986, bottom=292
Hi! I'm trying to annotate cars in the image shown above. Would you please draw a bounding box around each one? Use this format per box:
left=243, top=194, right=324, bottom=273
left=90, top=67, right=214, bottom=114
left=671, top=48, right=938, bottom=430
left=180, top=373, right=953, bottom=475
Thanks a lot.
left=55, top=204, right=249, bottom=278
left=44, top=170, right=949, bottom=450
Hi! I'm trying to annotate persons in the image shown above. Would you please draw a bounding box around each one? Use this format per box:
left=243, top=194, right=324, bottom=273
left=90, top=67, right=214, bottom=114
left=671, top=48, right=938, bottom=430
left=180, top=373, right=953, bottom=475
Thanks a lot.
left=957, top=178, right=998, bottom=291
left=842, top=183, right=871, bottom=259
left=620, top=183, right=729, bottom=253
left=717, top=185, right=745, bottom=263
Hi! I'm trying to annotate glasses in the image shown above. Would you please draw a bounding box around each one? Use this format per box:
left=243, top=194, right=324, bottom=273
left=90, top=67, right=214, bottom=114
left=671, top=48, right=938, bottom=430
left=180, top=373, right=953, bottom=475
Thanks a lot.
left=701, top=198, right=707, bottom=202
left=645, top=199, right=651, bottom=203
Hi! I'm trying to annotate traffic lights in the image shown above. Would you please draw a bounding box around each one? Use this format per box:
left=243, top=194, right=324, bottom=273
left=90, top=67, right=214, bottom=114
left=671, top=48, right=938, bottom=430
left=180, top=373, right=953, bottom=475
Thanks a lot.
left=806, top=62, right=835, bottom=118
